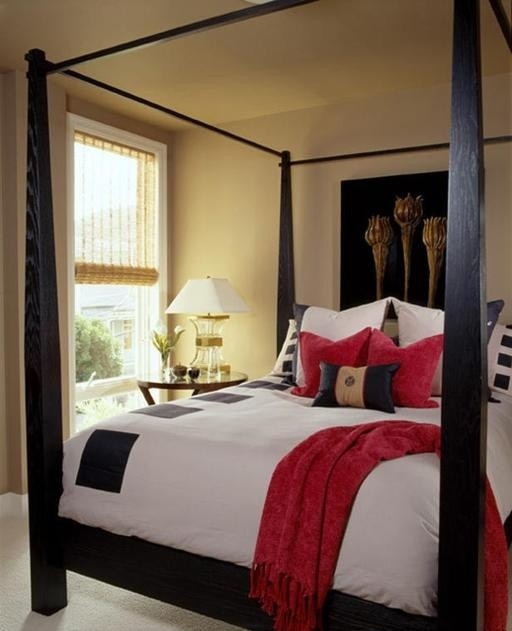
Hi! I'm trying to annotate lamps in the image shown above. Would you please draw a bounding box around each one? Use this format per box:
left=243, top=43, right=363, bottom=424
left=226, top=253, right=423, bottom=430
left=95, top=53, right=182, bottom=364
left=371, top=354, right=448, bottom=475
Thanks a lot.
left=162, top=276, right=249, bottom=376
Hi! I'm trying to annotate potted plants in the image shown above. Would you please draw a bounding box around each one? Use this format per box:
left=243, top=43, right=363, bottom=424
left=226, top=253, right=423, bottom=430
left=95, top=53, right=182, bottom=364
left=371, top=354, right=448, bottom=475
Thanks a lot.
left=151, top=329, right=187, bottom=377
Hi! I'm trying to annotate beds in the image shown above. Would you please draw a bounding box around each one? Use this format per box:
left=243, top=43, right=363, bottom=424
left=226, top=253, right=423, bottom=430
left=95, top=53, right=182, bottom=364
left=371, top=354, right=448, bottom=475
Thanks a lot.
left=62, top=372, right=512, bottom=631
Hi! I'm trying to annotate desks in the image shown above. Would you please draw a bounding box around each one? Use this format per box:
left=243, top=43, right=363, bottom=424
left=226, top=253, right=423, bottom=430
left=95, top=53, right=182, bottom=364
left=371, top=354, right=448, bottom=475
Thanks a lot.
left=136, top=370, right=248, bottom=405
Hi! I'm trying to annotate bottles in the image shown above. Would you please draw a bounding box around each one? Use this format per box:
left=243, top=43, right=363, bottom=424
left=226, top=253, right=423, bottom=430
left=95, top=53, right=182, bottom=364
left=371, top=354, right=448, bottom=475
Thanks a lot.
left=206, top=345, right=221, bottom=379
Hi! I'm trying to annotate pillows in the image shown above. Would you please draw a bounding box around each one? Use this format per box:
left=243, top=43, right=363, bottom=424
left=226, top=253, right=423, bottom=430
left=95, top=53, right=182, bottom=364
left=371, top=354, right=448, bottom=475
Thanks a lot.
left=272, top=297, right=512, bottom=414
